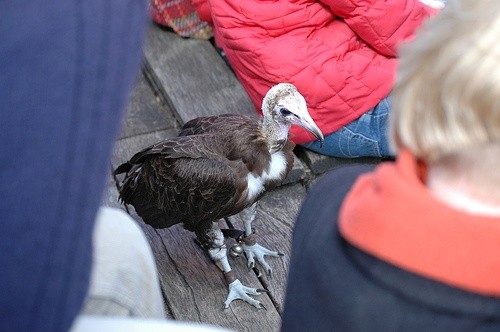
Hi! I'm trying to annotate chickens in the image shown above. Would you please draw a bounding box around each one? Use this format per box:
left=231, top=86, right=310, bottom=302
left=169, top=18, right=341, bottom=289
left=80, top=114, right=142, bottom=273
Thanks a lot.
left=111, top=83, right=325, bottom=312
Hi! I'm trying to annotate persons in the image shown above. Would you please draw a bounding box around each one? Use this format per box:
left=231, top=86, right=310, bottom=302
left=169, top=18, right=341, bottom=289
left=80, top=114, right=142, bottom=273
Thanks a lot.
left=280, top=1, right=500, bottom=332
left=0, top=0, right=443, bottom=332
